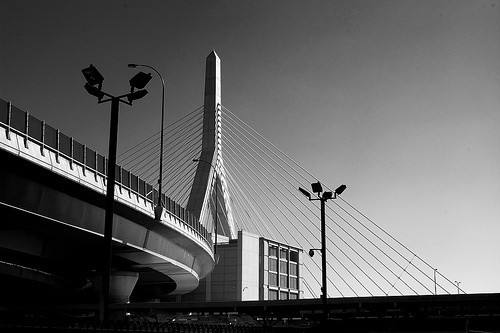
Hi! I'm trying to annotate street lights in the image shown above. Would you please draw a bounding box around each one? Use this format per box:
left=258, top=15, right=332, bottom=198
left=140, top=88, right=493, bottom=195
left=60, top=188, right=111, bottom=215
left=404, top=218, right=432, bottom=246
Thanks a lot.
left=298, top=180, right=346, bottom=321
left=192, top=159, right=219, bottom=256
left=82, top=64, right=154, bottom=330
left=127, top=63, right=167, bottom=207
